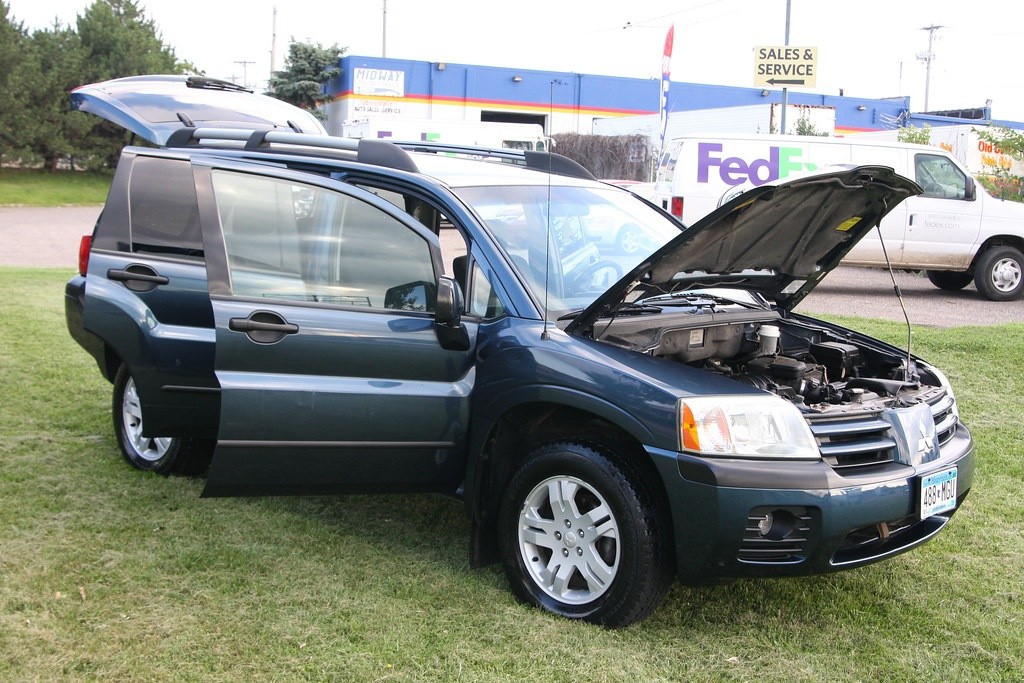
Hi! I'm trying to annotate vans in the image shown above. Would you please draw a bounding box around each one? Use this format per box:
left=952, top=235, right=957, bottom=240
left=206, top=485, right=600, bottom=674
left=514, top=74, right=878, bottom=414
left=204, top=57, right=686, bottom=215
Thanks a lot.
left=654, top=129, right=1023, bottom=303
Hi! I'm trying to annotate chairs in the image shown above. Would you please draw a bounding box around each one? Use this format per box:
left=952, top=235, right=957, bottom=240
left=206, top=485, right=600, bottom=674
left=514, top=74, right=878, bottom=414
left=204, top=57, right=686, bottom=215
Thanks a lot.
left=452, top=220, right=533, bottom=304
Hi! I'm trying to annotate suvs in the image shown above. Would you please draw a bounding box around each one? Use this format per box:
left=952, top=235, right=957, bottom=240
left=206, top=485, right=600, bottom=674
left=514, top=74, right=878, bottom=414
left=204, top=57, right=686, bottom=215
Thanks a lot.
left=63, top=73, right=975, bottom=633
